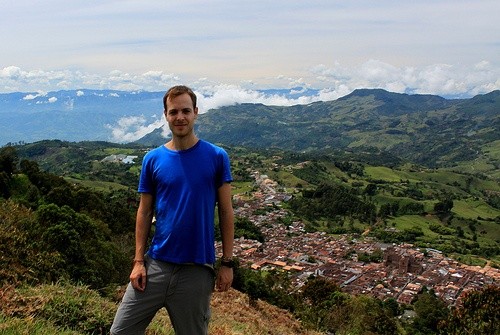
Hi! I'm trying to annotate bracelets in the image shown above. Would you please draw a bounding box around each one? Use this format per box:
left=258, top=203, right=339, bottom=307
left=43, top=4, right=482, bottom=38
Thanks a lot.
left=130, top=259, right=143, bottom=263
left=220, top=255, right=233, bottom=260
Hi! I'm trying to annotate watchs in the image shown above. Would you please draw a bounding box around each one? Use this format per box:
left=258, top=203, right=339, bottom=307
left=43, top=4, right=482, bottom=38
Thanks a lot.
left=220, top=260, right=236, bottom=268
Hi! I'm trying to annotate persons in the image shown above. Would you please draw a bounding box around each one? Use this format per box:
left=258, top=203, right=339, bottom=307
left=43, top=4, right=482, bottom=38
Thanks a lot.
left=110, top=86, right=234, bottom=335
